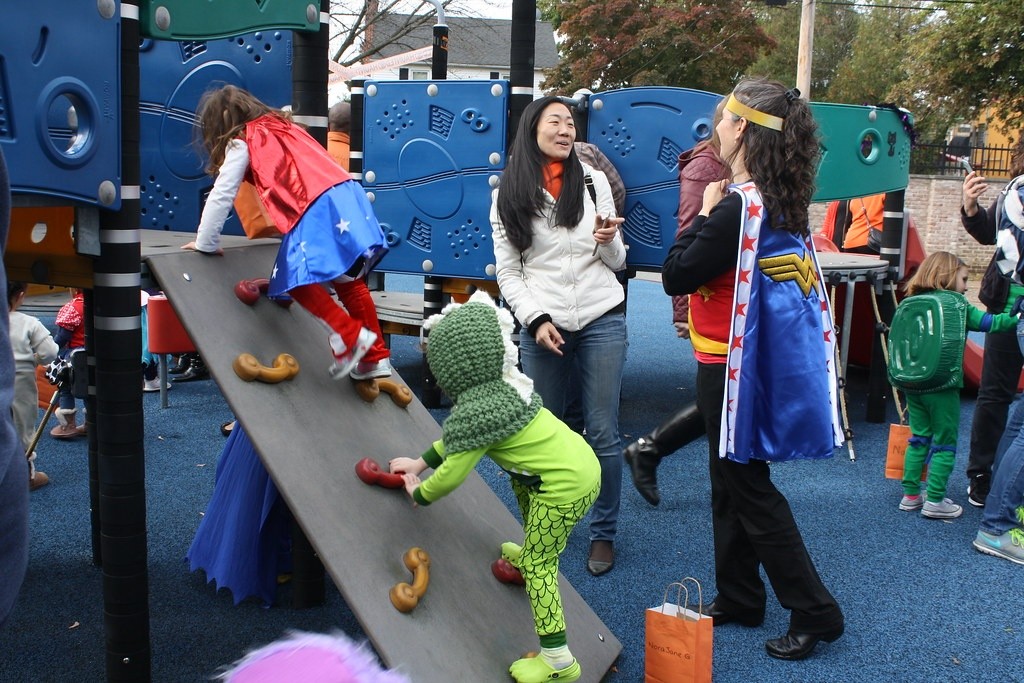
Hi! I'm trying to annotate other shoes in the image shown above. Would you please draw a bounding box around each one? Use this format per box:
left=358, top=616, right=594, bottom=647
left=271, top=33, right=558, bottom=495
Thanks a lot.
left=587, top=539, right=614, bottom=577
left=221, top=422, right=233, bottom=434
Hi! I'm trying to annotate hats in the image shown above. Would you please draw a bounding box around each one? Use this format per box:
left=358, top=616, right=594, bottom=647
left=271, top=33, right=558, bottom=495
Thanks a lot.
left=421, top=289, right=543, bottom=458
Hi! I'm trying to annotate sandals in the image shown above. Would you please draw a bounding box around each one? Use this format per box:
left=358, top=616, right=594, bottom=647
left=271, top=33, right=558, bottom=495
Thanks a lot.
left=500, top=541, right=522, bottom=567
left=508, top=652, right=582, bottom=683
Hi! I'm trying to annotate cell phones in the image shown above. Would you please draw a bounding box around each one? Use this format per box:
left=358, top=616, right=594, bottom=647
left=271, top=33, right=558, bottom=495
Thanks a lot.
left=960, top=160, right=978, bottom=187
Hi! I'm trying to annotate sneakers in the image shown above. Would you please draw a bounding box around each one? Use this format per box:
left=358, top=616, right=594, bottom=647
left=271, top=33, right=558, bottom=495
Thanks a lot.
left=898, top=496, right=925, bottom=511
left=1015, top=506, right=1024, bottom=525
left=349, top=356, right=393, bottom=381
left=328, top=327, right=377, bottom=380
left=921, top=498, right=963, bottom=518
left=966, top=472, right=991, bottom=507
left=973, top=528, right=1024, bottom=566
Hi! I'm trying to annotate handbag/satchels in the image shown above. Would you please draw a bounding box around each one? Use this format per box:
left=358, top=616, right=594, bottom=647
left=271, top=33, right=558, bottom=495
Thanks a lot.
left=886, top=408, right=928, bottom=482
left=868, top=228, right=883, bottom=253
left=645, top=577, right=713, bottom=683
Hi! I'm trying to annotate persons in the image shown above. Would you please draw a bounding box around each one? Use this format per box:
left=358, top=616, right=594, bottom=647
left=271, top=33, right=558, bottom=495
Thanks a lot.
left=820, top=136, right=1024, bottom=567
left=390, top=303, right=601, bottom=683
left=8, top=280, right=207, bottom=489
left=625, top=95, right=734, bottom=505
left=661, top=80, right=845, bottom=660
left=490, top=95, right=629, bottom=575
left=180, top=84, right=392, bottom=380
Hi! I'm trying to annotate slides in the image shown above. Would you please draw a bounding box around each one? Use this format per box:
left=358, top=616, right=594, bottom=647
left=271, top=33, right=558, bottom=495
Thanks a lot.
left=896, top=284, right=1024, bottom=392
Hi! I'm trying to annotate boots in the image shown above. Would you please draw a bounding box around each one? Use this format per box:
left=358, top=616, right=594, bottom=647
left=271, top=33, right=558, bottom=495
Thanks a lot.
left=50, top=407, right=79, bottom=438
left=168, top=353, right=190, bottom=374
left=623, top=401, right=708, bottom=507
left=28, top=451, right=49, bottom=490
left=144, top=376, right=171, bottom=391
left=172, top=354, right=203, bottom=382
left=75, top=407, right=87, bottom=436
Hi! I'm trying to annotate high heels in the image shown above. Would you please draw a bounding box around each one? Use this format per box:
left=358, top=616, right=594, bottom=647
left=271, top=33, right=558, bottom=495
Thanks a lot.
left=765, top=610, right=844, bottom=659
left=686, top=599, right=766, bottom=628
